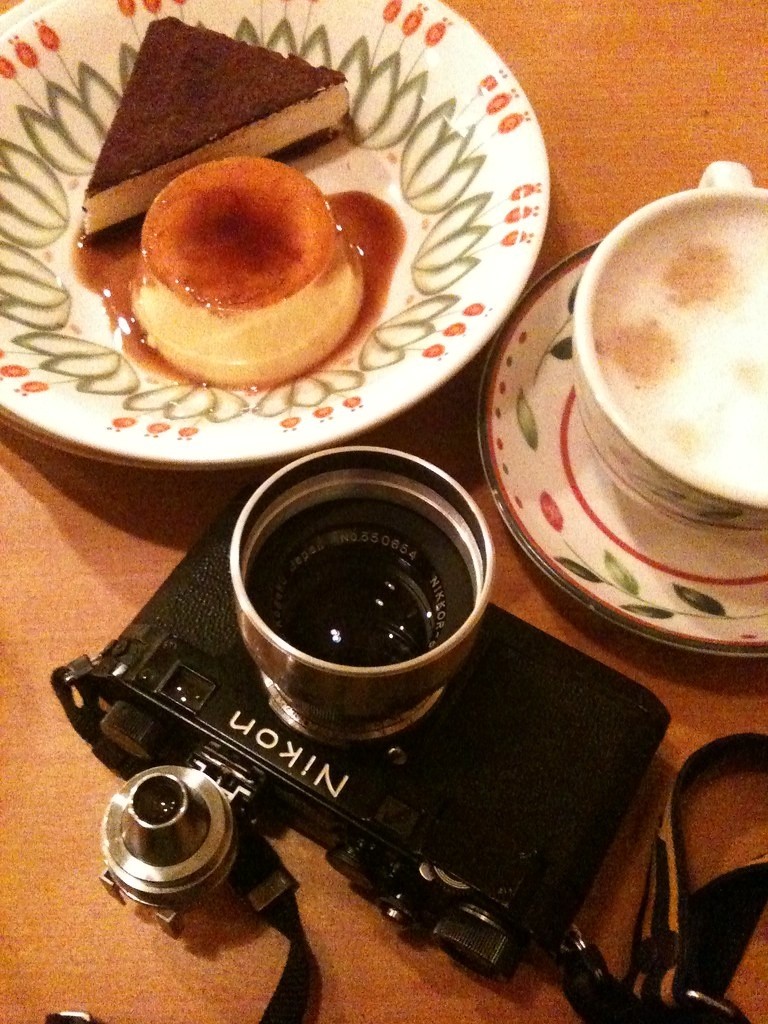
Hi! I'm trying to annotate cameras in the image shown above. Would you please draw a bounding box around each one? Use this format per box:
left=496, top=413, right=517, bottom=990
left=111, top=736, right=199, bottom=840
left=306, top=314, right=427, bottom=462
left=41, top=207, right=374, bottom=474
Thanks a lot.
left=82, top=447, right=670, bottom=982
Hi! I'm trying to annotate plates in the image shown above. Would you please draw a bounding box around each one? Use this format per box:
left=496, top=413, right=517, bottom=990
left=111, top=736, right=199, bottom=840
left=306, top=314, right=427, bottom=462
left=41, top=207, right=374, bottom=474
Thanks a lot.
left=0, top=0, right=551, bottom=468
left=477, top=237, right=768, bottom=657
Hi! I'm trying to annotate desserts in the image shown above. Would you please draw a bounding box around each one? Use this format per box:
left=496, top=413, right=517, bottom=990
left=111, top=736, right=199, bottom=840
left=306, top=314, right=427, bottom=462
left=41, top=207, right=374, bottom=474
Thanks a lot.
left=130, top=156, right=361, bottom=388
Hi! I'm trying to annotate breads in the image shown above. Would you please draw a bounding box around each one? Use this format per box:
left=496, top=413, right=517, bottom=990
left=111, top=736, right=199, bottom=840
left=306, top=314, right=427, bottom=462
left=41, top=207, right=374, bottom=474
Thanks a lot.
left=83, top=16, right=349, bottom=237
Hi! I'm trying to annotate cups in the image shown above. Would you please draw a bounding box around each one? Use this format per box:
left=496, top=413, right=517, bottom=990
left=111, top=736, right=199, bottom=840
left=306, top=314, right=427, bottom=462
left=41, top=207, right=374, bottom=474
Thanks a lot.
left=571, top=160, right=768, bottom=540
